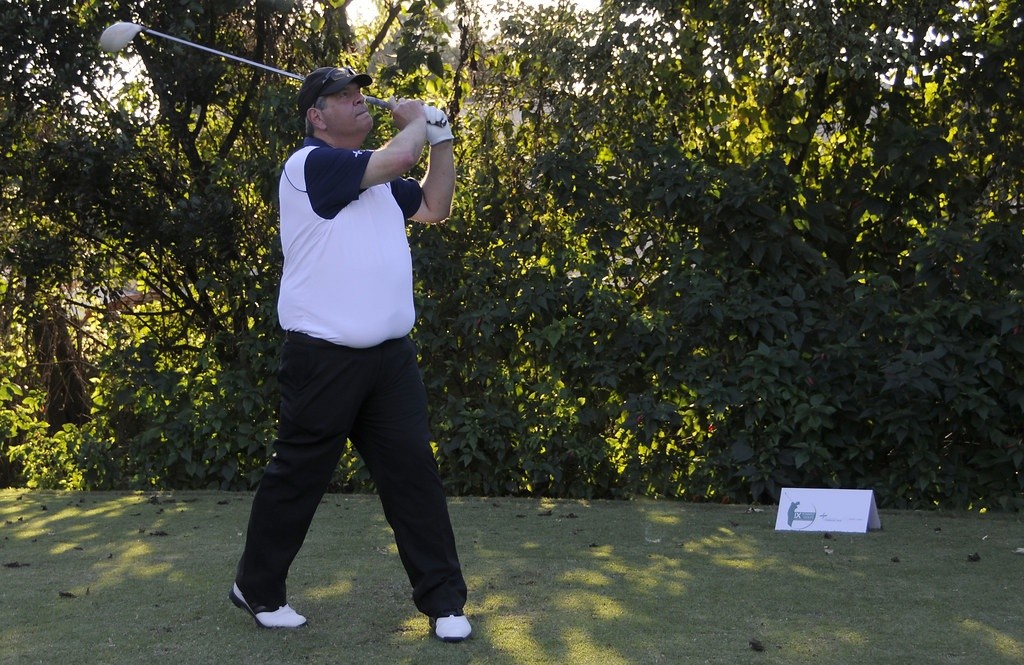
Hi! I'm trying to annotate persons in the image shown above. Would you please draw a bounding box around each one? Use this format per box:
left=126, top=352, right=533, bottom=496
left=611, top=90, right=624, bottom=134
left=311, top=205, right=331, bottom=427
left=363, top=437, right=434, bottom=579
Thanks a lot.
left=227, top=66, right=474, bottom=641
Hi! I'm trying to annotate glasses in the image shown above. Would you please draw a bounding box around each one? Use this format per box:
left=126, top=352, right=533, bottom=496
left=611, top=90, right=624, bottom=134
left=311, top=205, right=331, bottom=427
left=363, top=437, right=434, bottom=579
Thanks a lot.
left=314, top=67, right=357, bottom=108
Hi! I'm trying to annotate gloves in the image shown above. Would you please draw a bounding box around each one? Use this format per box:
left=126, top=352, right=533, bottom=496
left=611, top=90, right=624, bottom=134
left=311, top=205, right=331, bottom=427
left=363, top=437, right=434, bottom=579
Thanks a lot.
left=425, top=104, right=453, bottom=145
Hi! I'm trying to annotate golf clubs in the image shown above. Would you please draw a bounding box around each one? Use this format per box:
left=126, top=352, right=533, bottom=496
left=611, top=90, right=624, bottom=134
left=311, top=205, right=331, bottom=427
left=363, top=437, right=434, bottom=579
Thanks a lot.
left=97, top=20, right=447, bottom=131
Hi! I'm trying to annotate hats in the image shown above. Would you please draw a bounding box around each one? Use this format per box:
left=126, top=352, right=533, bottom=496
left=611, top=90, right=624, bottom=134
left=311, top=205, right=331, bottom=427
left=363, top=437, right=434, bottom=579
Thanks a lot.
left=299, top=67, right=373, bottom=117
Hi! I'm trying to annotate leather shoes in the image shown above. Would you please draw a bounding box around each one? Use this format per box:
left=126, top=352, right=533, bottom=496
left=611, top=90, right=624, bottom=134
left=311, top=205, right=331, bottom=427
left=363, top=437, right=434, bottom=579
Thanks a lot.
left=432, top=609, right=473, bottom=641
left=228, top=578, right=307, bottom=630
